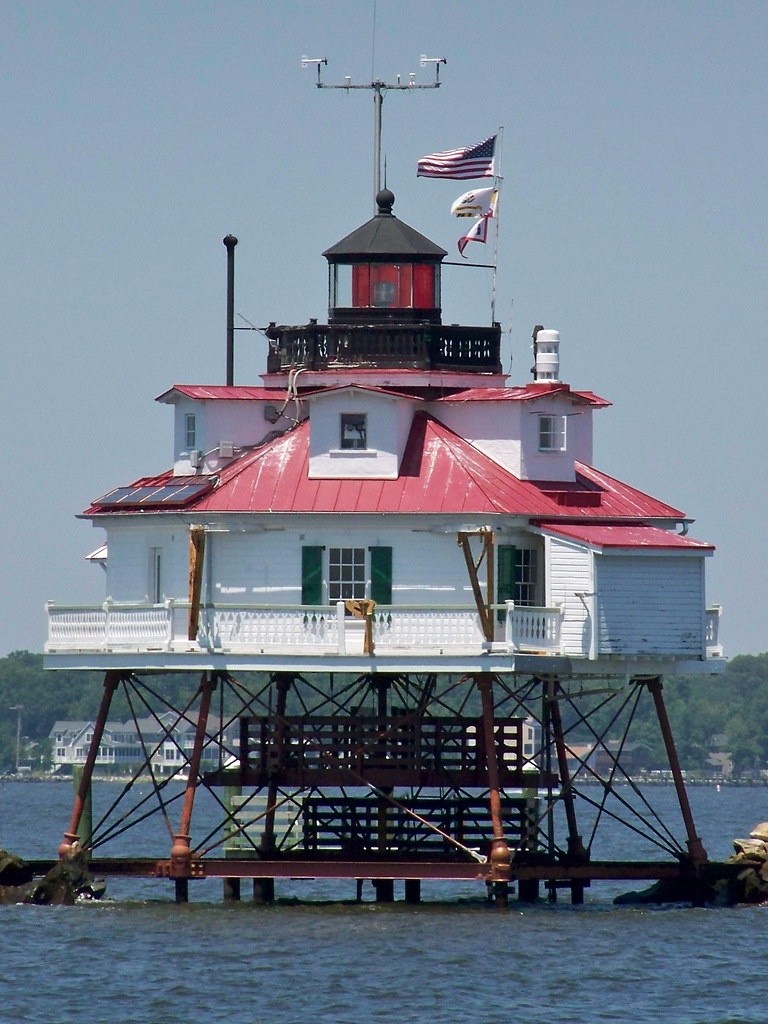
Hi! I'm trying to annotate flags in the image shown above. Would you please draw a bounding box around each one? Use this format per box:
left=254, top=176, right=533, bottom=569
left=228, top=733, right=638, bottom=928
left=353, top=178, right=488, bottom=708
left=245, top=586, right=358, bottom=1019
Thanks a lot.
left=450, top=187, right=499, bottom=218
left=417, top=134, right=495, bottom=179
left=457, top=210, right=494, bottom=258
